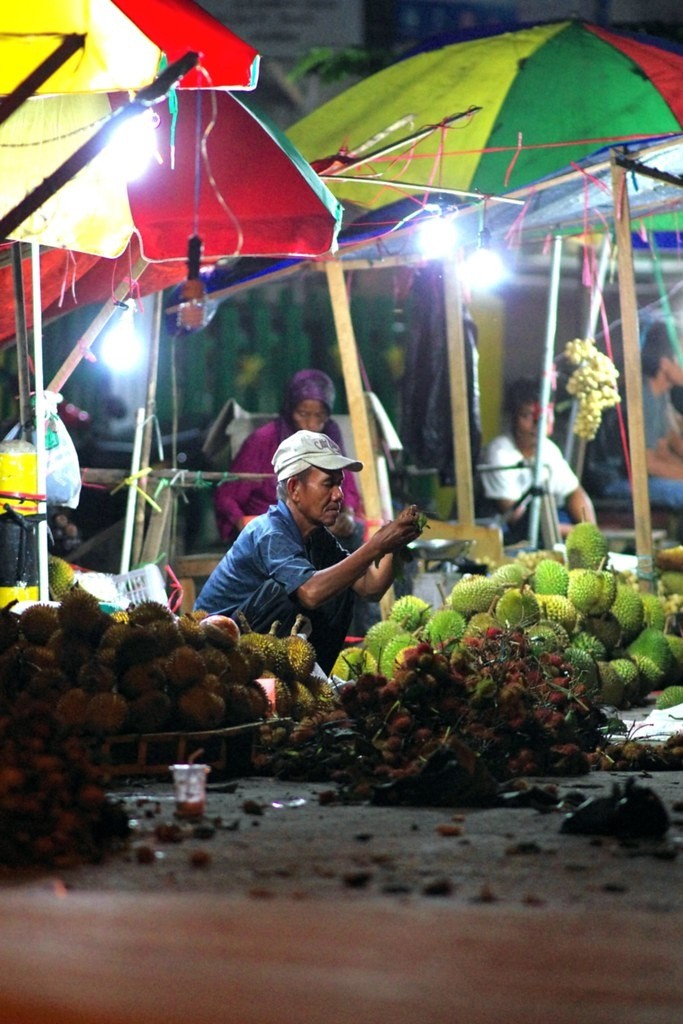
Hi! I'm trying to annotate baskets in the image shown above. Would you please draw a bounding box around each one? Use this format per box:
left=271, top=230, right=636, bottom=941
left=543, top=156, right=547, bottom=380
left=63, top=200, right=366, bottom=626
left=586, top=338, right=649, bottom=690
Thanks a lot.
left=113, top=563, right=167, bottom=607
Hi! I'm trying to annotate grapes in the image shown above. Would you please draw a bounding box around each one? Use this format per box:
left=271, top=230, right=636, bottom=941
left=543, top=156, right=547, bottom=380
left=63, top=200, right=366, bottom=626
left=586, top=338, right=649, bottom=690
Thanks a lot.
left=563, top=337, right=622, bottom=442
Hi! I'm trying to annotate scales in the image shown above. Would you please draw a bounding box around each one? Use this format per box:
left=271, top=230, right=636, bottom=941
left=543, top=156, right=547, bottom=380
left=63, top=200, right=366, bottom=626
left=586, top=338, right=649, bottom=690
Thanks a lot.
left=407, top=538, right=477, bottom=606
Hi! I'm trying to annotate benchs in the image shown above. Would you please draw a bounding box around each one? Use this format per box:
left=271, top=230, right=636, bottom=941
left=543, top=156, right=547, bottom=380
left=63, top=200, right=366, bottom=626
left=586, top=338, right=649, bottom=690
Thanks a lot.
left=173, top=553, right=227, bottom=617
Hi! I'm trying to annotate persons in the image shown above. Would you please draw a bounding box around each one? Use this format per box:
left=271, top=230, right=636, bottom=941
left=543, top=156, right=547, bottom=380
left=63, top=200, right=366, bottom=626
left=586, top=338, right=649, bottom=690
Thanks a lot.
left=584, top=324, right=683, bottom=512
left=216, top=371, right=365, bottom=561
left=193, top=429, right=424, bottom=678
left=455, top=378, right=598, bottom=549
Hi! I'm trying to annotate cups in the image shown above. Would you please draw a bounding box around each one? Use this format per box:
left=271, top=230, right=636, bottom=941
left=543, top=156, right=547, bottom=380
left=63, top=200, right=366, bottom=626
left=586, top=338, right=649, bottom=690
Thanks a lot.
left=170, top=763, right=211, bottom=815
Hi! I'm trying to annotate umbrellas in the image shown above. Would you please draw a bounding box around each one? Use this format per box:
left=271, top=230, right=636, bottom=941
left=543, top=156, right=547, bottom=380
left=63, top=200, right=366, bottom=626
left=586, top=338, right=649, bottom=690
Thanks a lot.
left=0, top=0, right=345, bottom=607
left=162, top=21, right=682, bottom=550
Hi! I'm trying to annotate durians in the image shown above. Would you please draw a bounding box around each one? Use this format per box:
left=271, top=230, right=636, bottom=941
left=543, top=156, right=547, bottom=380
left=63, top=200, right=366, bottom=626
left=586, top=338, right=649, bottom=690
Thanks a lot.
left=330, top=509, right=683, bottom=712
left=0, top=556, right=331, bottom=739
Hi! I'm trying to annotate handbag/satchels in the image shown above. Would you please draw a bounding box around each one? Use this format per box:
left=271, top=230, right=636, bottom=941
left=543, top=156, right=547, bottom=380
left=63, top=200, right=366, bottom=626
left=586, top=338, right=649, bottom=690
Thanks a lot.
left=18, top=387, right=83, bottom=509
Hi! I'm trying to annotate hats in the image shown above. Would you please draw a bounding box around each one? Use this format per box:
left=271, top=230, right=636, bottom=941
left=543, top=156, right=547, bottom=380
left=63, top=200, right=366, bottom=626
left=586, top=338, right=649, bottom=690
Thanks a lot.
left=271, top=430, right=362, bottom=483
